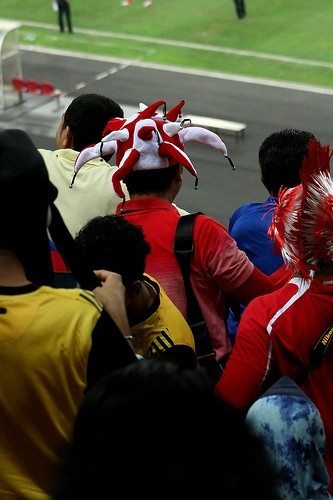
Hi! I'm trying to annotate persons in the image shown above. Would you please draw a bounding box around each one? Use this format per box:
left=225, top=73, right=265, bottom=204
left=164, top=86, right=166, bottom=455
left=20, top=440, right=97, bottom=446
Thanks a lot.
left=54, top=0, right=75, bottom=35
left=1, top=93, right=333, bottom=500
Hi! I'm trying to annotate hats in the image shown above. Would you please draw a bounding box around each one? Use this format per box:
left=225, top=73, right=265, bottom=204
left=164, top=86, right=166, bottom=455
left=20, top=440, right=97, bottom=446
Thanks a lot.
left=69, top=100, right=236, bottom=215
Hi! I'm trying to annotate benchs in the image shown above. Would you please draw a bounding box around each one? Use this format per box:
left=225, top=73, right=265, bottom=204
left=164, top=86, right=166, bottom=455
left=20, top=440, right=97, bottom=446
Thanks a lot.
left=184, top=114, right=247, bottom=143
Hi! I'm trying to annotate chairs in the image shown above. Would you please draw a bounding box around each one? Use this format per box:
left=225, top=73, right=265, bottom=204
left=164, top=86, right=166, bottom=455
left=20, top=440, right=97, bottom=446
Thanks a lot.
left=10, top=76, right=66, bottom=113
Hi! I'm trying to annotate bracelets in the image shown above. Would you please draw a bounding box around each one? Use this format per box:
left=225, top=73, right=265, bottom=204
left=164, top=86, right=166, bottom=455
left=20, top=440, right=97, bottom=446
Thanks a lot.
left=126, top=335, right=135, bottom=341
